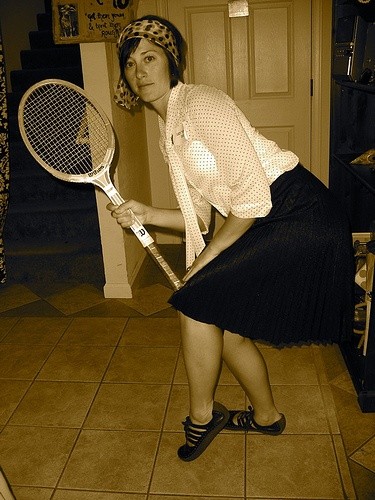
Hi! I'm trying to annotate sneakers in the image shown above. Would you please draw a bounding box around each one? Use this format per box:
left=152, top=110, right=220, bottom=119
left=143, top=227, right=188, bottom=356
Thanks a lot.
left=224, top=406, right=286, bottom=435
left=178, top=401, right=230, bottom=462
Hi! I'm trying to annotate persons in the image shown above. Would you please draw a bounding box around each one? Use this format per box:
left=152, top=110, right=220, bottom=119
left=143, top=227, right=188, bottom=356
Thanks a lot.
left=106, top=15, right=355, bottom=462
